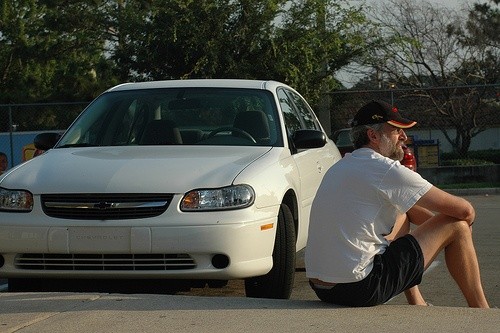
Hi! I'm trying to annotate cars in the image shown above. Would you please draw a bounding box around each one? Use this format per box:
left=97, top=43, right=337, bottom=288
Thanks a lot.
left=0, top=79, right=343, bottom=299
left=330, top=127, right=417, bottom=174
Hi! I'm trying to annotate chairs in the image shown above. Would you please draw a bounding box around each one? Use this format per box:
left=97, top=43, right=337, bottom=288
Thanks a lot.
left=133, top=119, right=183, bottom=144
left=180, top=129, right=209, bottom=145
left=232, top=109, right=273, bottom=147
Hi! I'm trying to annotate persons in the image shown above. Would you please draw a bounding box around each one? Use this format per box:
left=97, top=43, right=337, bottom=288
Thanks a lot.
left=303, top=98, right=491, bottom=308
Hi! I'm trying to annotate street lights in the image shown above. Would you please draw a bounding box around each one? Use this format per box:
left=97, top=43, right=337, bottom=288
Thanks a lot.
left=390, top=82, right=395, bottom=106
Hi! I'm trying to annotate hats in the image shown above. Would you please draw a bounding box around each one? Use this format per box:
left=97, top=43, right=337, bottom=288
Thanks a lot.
left=351, top=100, right=417, bottom=129
